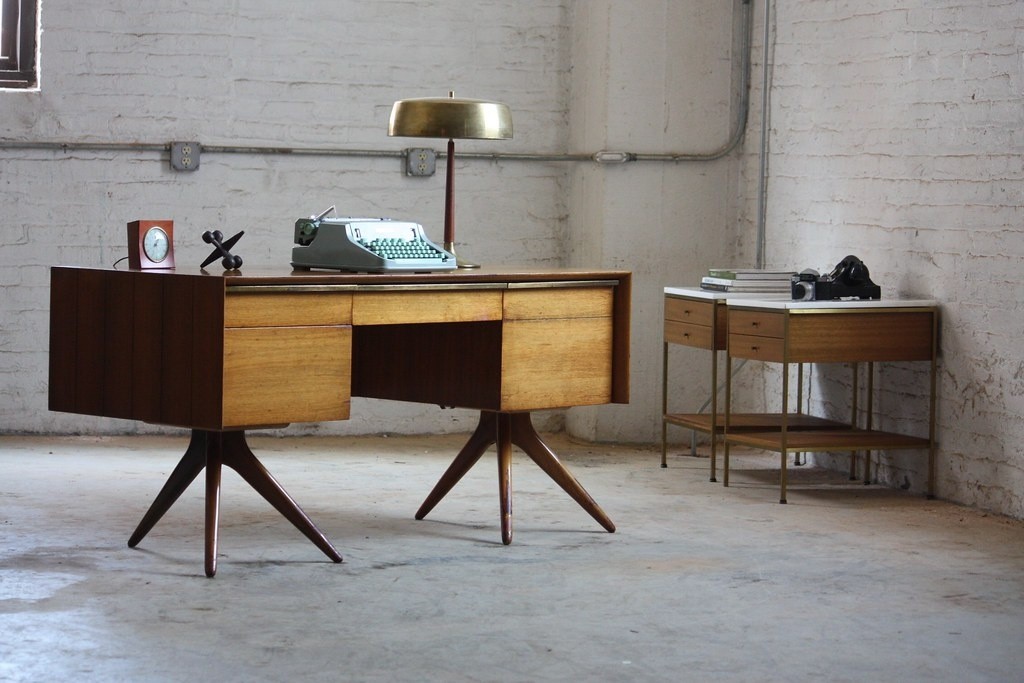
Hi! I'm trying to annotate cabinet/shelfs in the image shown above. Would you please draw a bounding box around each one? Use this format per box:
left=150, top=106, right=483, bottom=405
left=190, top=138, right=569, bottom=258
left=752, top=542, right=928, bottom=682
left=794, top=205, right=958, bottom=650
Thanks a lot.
left=41, top=265, right=635, bottom=579
left=722, top=299, right=940, bottom=505
left=662, top=284, right=864, bottom=480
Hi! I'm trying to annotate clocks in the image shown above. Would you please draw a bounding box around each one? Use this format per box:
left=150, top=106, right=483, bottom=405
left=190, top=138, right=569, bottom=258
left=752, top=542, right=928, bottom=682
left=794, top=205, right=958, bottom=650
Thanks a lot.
left=128, top=218, right=179, bottom=268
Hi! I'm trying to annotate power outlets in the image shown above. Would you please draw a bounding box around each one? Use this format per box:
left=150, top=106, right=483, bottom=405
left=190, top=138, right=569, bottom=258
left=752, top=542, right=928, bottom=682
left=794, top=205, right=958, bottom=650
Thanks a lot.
left=170, top=140, right=203, bottom=172
left=409, top=145, right=438, bottom=179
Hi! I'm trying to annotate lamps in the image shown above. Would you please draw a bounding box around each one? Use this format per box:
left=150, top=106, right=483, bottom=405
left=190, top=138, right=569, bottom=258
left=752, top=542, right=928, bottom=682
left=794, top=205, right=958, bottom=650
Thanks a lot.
left=388, top=89, right=513, bottom=269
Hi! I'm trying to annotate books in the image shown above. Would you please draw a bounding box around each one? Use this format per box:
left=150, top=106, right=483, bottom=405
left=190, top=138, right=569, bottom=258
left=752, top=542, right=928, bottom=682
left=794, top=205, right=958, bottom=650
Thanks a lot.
left=699, top=268, right=799, bottom=293
